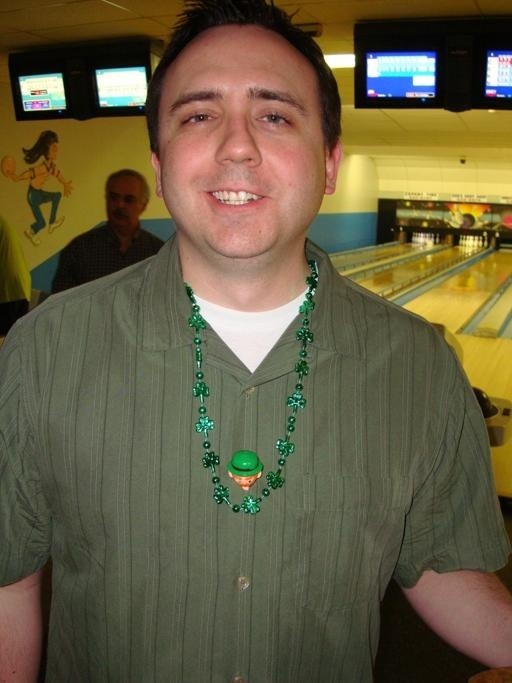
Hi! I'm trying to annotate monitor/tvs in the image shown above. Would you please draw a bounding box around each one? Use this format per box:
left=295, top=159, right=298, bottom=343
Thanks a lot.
left=363, top=49, right=441, bottom=102
left=18, top=71, right=69, bottom=113
left=93, top=65, right=150, bottom=108
left=482, top=48, right=512, bottom=104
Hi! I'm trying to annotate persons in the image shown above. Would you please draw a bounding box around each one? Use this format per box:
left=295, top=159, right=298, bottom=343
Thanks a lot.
left=0, top=0, right=512, bottom=683
left=50, top=168, right=165, bottom=293
left=0, top=210, right=32, bottom=337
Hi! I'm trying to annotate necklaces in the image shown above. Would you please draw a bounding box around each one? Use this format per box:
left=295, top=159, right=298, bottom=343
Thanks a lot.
left=182, top=259, right=319, bottom=515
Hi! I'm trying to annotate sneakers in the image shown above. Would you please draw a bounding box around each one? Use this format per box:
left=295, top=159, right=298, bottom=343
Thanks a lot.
left=49, top=216, right=65, bottom=233
left=24, top=225, right=40, bottom=246
